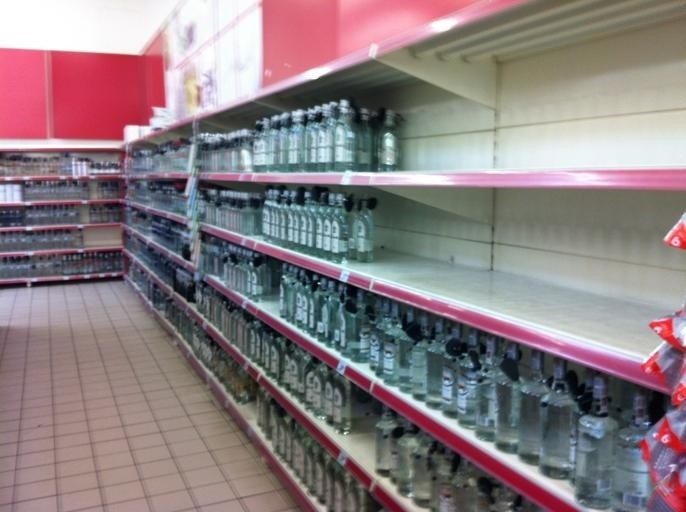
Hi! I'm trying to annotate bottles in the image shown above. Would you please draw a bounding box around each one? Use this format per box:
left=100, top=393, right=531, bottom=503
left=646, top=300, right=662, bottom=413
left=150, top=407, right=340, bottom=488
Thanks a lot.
left=0, top=153, right=124, bottom=279
left=219, top=242, right=658, bottom=511
left=197, top=98, right=400, bottom=264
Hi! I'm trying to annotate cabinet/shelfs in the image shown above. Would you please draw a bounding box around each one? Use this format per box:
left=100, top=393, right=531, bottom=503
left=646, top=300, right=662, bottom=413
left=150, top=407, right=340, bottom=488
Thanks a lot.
left=120, top=1, right=686, bottom=512
left=1, top=140, right=119, bottom=283
left=5, top=46, right=121, bottom=139
